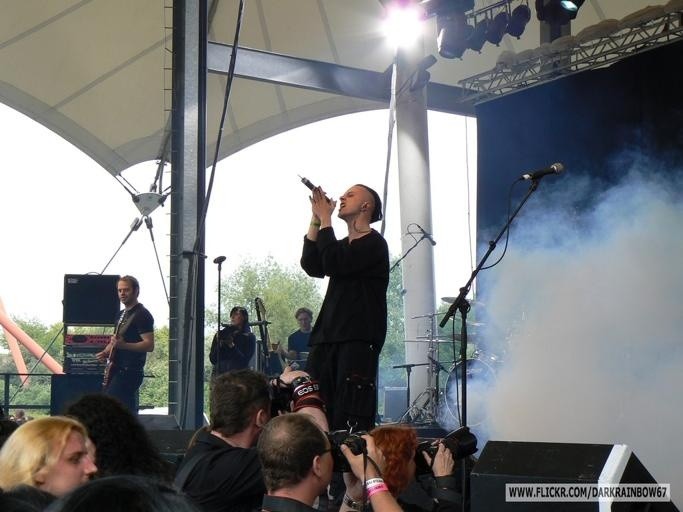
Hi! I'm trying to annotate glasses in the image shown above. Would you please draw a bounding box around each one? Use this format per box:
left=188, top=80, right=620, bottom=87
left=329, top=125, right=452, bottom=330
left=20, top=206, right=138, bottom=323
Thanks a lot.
left=322, top=438, right=338, bottom=455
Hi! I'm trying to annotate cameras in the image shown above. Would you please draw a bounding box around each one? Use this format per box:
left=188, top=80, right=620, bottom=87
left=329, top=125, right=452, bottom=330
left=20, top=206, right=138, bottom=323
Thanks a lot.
left=271, top=386, right=295, bottom=418
left=416, top=426, right=478, bottom=476
left=324, top=430, right=368, bottom=472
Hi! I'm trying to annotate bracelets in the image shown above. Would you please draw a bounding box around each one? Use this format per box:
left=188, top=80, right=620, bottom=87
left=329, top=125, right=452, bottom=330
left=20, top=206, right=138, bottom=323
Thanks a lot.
left=309, top=219, right=321, bottom=229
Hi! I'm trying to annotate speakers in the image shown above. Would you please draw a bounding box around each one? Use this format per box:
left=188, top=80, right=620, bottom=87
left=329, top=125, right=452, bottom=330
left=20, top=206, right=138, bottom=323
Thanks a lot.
left=51, top=374, right=105, bottom=416
left=471, top=442, right=678, bottom=511
left=63, top=275, right=120, bottom=325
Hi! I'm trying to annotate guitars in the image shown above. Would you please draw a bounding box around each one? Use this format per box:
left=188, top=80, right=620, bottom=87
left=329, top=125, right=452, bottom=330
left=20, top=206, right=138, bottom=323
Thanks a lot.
left=102, top=310, right=127, bottom=393
left=254, top=297, right=287, bottom=370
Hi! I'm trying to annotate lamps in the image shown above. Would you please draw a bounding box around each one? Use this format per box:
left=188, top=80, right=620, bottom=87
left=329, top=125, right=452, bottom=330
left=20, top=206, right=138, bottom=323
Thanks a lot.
left=417, top=0, right=584, bottom=59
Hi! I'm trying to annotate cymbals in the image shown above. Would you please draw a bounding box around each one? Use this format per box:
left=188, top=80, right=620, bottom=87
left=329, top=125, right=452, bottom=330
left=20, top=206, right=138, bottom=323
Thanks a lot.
left=441, top=296, right=472, bottom=305
left=402, top=339, right=452, bottom=343
left=448, top=333, right=478, bottom=345
left=413, top=336, right=450, bottom=339
left=413, top=312, right=450, bottom=318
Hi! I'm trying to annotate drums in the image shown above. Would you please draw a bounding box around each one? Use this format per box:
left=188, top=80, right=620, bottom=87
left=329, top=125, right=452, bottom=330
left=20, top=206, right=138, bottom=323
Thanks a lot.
left=444, top=354, right=507, bottom=428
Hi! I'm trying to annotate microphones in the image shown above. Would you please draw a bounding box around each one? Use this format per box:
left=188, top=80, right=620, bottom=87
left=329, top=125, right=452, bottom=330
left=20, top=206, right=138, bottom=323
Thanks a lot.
left=417, top=225, right=436, bottom=246
left=522, top=163, right=563, bottom=180
left=301, top=177, right=330, bottom=204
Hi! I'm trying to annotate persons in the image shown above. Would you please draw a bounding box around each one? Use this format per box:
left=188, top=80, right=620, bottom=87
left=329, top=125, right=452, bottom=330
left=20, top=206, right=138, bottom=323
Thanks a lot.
left=207, top=306, right=256, bottom=383
left=269, top=308, right=315, bottom=372
left=0, top=370, right=477, bottom=512
left=95, top=274, right=154, bottom=422
left=300, top=184, right=389, bottom=429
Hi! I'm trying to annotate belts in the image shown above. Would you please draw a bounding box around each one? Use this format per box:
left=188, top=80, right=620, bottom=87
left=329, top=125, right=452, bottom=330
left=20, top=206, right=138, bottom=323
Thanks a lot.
left=119, top=367, right=139, bottom=370
